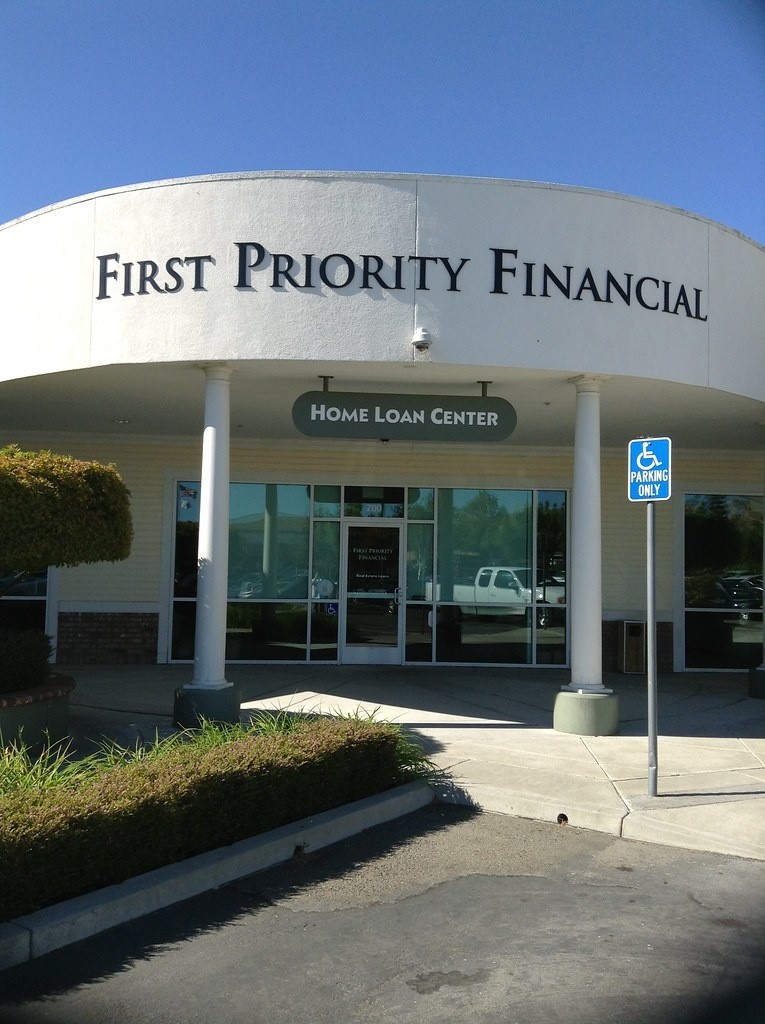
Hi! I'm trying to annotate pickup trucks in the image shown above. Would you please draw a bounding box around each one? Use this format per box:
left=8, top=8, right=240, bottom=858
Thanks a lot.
left=425, top=566, right=566, bottom=629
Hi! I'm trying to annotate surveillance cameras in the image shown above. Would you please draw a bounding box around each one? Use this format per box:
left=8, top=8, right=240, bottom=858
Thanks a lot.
left=411, top=327, right=433, bottom=353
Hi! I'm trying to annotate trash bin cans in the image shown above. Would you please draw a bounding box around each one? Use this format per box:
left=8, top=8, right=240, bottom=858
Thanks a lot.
left=617, top=619, right=646, bottom=675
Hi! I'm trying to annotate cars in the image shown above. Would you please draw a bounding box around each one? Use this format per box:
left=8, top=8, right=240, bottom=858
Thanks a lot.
left=681, top=568, right=764, bottom=620
